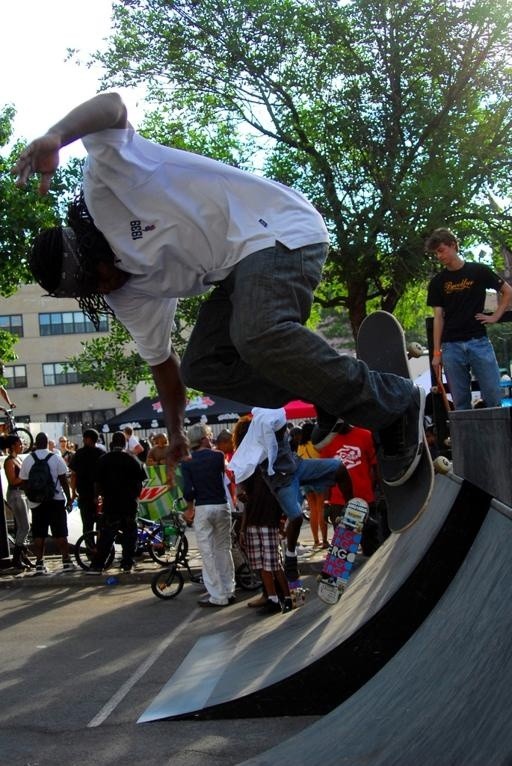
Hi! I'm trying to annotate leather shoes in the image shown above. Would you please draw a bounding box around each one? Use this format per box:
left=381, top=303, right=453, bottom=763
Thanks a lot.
left=197, top=599, right=228, bottom=607
left=227, top=596, right=236, bottom=605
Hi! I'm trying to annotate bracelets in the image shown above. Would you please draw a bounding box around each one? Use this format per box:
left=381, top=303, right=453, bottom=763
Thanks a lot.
left=433, top=352, right=441, bottom=356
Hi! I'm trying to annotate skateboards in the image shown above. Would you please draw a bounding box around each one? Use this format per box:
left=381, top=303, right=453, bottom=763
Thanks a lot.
left=354, top=310, right=452, bottom=535
left=315, top=497, right=371, bottom=605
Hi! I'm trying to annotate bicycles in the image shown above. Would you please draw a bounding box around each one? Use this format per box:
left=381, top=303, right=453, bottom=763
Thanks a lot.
left=0, top=406, right=34, bottom=454
left=150, top=497, right=264, bottom=600
left=74, top=505, right=189, bottom=572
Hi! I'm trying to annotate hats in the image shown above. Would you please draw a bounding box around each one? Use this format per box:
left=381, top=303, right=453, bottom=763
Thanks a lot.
left=188, top=424, right=211, bottom=450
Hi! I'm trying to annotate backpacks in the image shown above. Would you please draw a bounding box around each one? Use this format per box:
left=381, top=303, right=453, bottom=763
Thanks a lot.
left=25, top=452, right=58, bottom=504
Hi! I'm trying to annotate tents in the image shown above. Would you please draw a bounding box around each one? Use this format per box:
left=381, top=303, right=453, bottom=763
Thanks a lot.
left=95, top=395, right=241, bottom=432
left=416, top=362, right=508, bottom=394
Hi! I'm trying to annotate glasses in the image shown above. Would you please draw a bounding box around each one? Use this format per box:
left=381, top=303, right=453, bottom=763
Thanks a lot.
left=60, top=440, right=66, bottom=442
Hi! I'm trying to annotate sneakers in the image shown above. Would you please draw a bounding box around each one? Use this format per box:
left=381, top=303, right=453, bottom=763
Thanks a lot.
left=62, top=560, right=76, bottom=571
left=283, top=554, right=299, bottom=582
left=85, top=567, right=101, bottom=576
left=35, top=561, right=49, bottom=575
left=23, top=558, right=35, bottom=568
left=255, top=598, right=283, bottom=618
left=121, top=565, right=131, bottom=575
left=249, top=595, right=268, bottom=608
left=375, top=386, right=426, bottom=488
left=11, top=559, right=31, bottom=569
left=282, top=597, right=293, bottom=613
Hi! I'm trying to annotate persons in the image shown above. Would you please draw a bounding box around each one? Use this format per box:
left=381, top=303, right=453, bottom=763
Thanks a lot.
left=180, top=423, right=237, bottom=607
left=230, top=416, right=293, bottom=615
left=123, top=427, right=144, bottom=457
left=58, top=436, right=76, bottom=468
left=3, top=435, right=36, bottom=570
left=48, top=439, right=62, bottom=457
left=288, top=427, right=301, bottom=452
left=68, top=428, right=108, bottom=561
left=84, top=432, right=143, bottom=577
left=10, top=94, right=424, bottom=489
left=423, top=229, right=511, bottom=411
left=294, top=422, right=331, bottom=550
left=317, top=417, right=381, bottom=557
left=226, top=400, right=354, bottom=581
left=19, top=432, right=74, bottom=574
left=147, top=433, right=169, bottom=465
